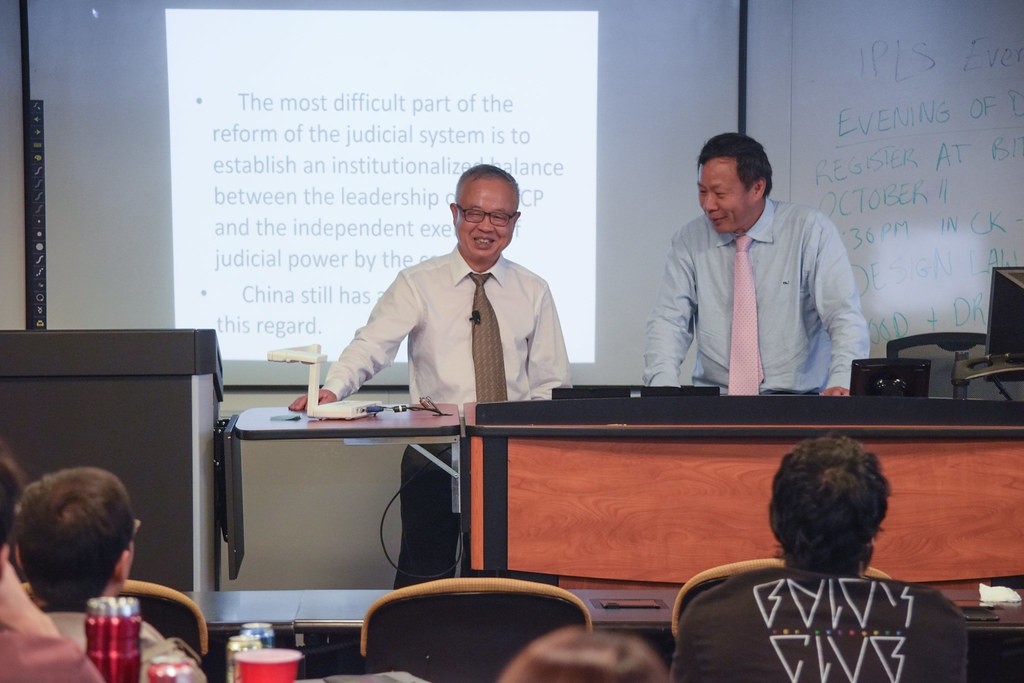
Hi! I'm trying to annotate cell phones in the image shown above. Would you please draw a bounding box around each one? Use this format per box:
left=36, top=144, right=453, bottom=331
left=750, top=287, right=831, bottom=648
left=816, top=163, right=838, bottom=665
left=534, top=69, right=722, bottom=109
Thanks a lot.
left=958, top=605, right=1000, bottom=622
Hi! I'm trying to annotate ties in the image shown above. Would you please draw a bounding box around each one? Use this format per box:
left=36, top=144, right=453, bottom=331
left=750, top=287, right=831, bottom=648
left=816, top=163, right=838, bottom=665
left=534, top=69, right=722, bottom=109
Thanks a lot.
left=728, top=236, right=764, bottom=396
left=468, top=272, right=508, bottom=402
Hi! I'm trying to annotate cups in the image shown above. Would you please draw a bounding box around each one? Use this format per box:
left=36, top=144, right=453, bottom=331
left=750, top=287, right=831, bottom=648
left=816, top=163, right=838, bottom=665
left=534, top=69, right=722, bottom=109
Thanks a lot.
left=233, top=648, right=303, bottom=683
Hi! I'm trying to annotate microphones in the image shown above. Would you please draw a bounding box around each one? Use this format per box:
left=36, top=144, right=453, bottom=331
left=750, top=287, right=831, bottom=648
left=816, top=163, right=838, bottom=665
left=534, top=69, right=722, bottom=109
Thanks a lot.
left=472, top=310, right=481, bottom=324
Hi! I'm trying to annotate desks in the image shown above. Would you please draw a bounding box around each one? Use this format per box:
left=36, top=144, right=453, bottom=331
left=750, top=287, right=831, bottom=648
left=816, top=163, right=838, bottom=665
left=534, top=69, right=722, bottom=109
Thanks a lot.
left=224, top=400, right=465, bottom=581
left=461, top=394, right=1024, bottom=589
left=300, top=586, right=1024, bottom=637
left=178, top=588, right=300, bottom=634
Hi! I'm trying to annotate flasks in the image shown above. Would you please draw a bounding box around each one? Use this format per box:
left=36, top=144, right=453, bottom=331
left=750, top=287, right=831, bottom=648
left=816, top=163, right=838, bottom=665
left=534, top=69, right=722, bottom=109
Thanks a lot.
left=78, top=597, right=144, bottom=683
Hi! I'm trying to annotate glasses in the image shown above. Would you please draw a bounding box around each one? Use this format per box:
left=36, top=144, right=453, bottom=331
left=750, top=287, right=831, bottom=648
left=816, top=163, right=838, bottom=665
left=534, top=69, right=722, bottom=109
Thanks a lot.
left=457, top=204, right=517, bottom=227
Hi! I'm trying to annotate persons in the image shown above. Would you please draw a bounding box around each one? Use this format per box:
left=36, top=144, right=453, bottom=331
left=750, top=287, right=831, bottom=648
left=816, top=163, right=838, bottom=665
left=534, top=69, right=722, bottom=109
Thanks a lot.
left=668, top=432, right=969, bottom=683
left=288, top=165, right=572, bottom=590
left=0, top=458, right=208, bottom=683
left=639, top=132, right=872, bottom=395
left=498, top=625, right=672, bottom=683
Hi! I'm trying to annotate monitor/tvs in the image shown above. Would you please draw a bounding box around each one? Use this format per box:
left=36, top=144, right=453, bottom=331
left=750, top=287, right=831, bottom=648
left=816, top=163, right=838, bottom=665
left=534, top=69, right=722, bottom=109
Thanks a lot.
left=849, top=358, right=931, bottom=397
left=984, top=267, right=1024, bottom=382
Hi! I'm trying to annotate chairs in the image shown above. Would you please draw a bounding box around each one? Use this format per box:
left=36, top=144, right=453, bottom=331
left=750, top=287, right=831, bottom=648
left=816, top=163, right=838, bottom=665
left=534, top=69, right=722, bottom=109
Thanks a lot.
left=119, top=580, right=210, bottom=653
left=887, top=332, right=986, bottom=399
left=670, top=557, right=893, bottom=648
left=357, top=574, right=595, bottom=683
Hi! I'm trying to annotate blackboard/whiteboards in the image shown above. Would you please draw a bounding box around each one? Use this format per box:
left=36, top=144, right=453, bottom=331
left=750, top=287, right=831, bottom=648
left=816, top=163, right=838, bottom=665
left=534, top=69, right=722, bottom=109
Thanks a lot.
left=735, top=0, right=1023, bottom=400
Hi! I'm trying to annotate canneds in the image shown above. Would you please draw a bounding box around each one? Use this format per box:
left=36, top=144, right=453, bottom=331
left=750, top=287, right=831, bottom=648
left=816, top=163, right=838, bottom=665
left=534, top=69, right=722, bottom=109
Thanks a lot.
left=225, top=623, right=275, bottom=683
left=146, top=655, right=199, bottom=683
